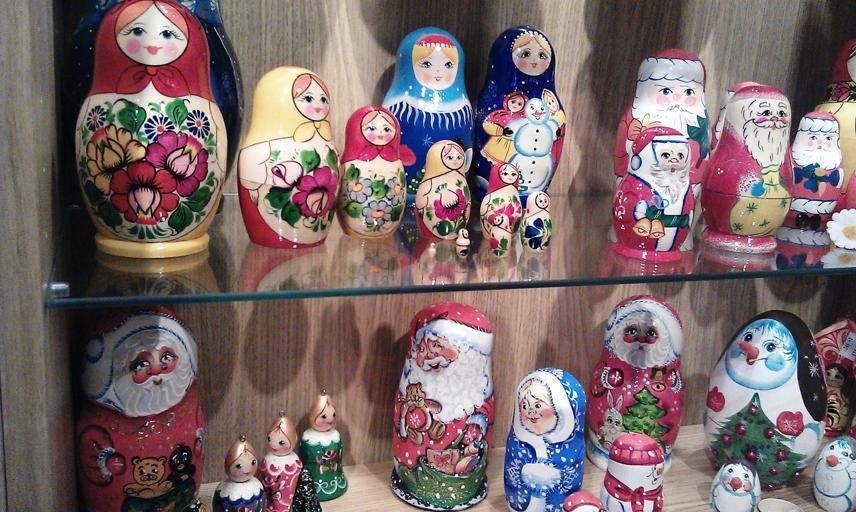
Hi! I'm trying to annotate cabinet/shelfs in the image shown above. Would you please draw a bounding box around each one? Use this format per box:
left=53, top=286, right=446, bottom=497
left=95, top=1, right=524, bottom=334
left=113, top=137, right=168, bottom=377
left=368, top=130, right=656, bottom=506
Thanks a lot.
left=0, top=0, right=856, bottom=512
